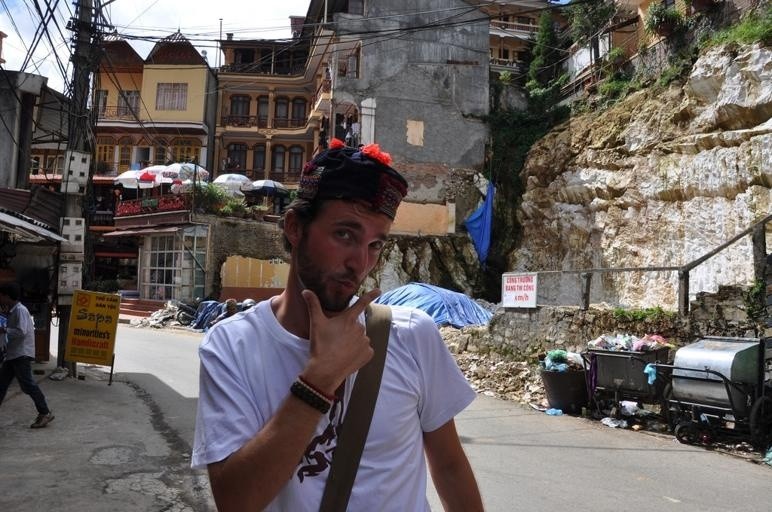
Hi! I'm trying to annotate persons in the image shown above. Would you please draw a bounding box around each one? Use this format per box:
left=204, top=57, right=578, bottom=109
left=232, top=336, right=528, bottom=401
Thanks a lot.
left=190, top=136, right=485, bottom=510
left=1, top=279, right=56, bottom=428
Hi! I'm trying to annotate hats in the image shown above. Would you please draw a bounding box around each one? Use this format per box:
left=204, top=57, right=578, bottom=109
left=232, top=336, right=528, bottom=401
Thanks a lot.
left=296, top=140, right=408, bottom=221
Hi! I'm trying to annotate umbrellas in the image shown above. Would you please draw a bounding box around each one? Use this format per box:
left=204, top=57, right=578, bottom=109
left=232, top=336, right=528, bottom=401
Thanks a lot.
left=114, top=159, right=289, bottom=205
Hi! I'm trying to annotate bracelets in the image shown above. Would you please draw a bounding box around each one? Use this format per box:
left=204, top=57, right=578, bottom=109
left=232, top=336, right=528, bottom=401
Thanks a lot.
left=288, top=373, right=334, bottom=415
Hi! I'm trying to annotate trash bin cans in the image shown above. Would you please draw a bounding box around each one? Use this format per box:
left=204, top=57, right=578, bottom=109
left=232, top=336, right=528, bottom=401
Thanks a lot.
left=580, top=345, right=676, bottom=432
left=540, top=368, right=593, bottom=416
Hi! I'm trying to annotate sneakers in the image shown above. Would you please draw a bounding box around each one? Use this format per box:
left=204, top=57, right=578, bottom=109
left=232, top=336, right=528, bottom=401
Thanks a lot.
left=31, top=412, right=54, bottom=428
